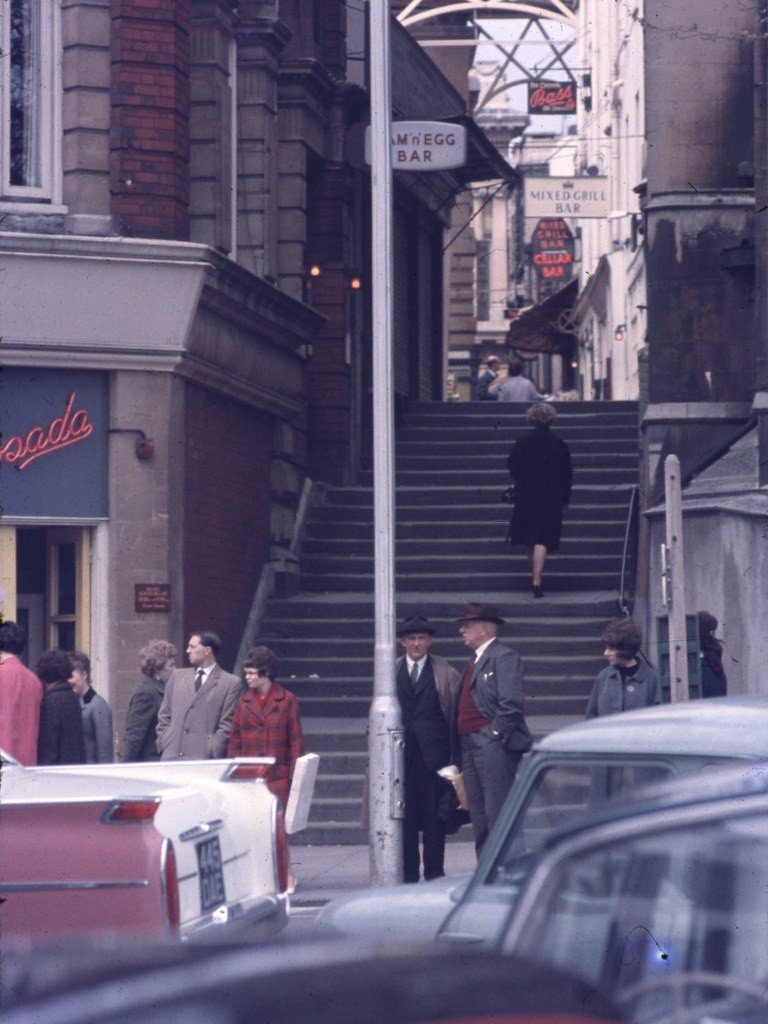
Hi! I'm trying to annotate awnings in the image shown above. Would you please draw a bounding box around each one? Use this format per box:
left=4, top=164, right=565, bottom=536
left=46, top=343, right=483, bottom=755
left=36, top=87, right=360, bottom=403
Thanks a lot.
left=506, top=276, right=577, bottom=351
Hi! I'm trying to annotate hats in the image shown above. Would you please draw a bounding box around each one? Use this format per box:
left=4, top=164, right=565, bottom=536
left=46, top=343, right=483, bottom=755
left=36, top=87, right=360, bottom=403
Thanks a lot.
left=456, top=602, right=506, bottom=625
left=395, top=617, right=437, bottom=640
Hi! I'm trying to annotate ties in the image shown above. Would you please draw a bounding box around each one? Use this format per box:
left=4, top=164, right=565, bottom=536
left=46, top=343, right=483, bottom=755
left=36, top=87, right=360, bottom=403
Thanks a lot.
left=470, top=653, right=477, bottom=663
left=194, top=669, right=205, bottom=692
left=410, top=661, right=418, bottom=686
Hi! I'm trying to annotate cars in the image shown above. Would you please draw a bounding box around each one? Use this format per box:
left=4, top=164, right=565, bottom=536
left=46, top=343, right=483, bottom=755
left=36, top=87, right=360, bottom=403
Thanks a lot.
left=0, top=693, right=767, bottom=1023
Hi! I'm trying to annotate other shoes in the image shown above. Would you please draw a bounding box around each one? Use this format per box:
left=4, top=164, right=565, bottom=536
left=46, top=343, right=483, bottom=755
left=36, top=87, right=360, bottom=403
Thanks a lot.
left=533, top=584, right=544, bottom=599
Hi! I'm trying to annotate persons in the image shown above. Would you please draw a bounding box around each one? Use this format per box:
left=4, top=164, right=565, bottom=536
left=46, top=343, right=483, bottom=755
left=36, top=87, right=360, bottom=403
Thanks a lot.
left=508, top=401, right=572, bottom=599
left=452, top=603, right=532, bottom=883
left=581, top=617, right=661, bottom=806
left=694, top=610, right=727, bottom=698
left=395, top=616, right=462, bottom=886
left=0, top=621, right=306, bottom=895
left=447, top=354, right=578, bottom=400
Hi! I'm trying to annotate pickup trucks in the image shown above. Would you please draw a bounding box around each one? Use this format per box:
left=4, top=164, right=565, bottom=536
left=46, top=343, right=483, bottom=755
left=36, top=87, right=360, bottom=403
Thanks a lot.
left=1, top=749, right=299, bottom=943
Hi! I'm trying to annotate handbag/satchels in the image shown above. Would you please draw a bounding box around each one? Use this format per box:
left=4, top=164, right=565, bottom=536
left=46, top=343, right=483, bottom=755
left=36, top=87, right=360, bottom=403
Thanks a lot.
left=502, top=487, right=516, bottom=504
